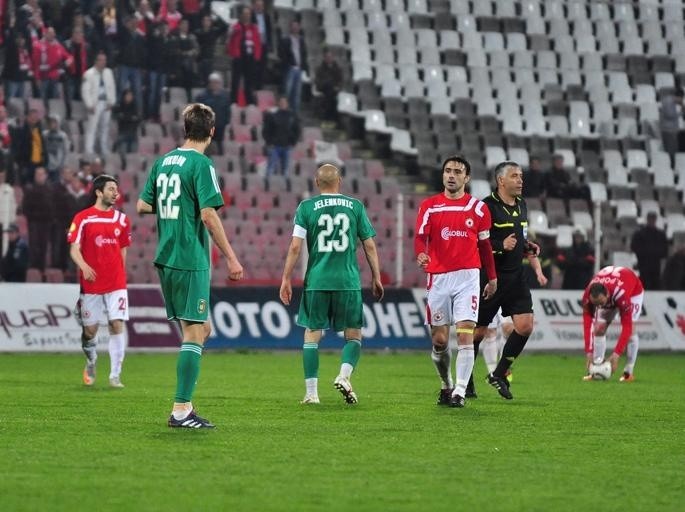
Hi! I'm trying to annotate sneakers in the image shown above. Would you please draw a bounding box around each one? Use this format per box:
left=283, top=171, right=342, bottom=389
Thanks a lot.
left=582, top=374, right=593, bottom=382
left=108, top=374, right=124, bottom=388
left=83, top=363, right=96, bottom=385
left=618, top=371, right=634, bottom=383
left=298, top=396, right=321, bottom=405
left=435, top=373, right=477, bottom=408
left=168, top=406, right=216, bottom=430
left=484, top=369, right=515, bottom=401
left=333, top=374, right=358, bottom=405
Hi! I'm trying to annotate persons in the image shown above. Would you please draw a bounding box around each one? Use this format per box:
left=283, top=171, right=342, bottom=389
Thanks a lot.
left=278, top=163, right=387, bottom=403
left=661, top=88, right=684, bottom=158
left=580, top=265, right=647, bottom=382
left=0, top=0, right=352, bottom=184
left=522, top=153, right=685, bottom=290
left=482, top=244, right=551, bottom=382
left=410, top=155, right=499, bottom=408
left=136, top=102, right=244, bottom=429
left=463, top=161, right=541, bottom=400
left=65, top=173, right=133, bottom=389
left=0, top=159, right=123, bottom=290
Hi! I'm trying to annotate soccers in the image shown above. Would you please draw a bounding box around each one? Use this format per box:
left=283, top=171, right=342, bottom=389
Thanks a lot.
left=589, top=358, right=612, bottom=381
